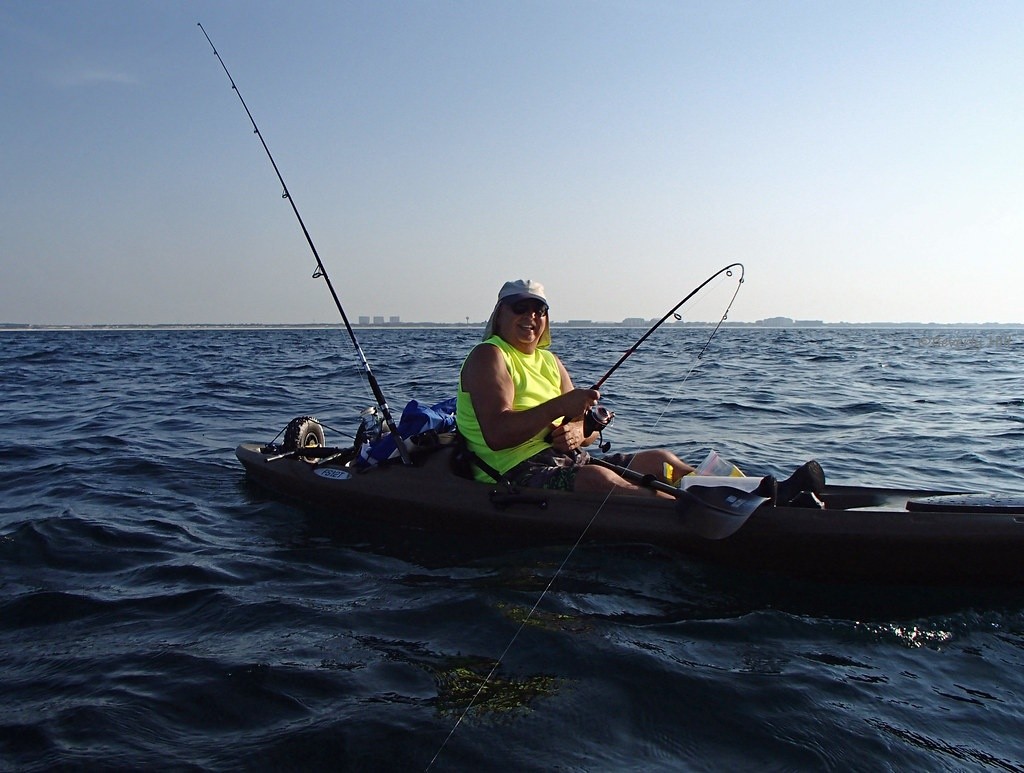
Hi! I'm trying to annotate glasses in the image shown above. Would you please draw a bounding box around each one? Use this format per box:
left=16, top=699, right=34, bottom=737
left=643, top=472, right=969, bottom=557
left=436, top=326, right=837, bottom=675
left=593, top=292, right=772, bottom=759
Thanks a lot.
left=503, top=301, right=547, bottom=317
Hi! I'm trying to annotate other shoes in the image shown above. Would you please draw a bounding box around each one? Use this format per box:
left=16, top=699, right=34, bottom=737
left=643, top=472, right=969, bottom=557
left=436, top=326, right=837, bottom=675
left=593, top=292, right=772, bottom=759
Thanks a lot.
left=797, top=460, right=827, bottom=507
left=755, top=475, right=777, bottom=507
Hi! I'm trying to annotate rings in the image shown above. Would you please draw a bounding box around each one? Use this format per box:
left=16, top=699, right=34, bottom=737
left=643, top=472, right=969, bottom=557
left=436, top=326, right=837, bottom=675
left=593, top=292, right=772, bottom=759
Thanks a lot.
left=570, top=439, right=572, bottom=446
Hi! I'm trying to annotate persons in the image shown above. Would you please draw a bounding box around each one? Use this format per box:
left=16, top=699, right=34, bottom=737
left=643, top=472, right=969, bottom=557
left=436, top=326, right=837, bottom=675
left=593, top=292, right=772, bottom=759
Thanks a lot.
left=459, top=281, right=718, bottom=502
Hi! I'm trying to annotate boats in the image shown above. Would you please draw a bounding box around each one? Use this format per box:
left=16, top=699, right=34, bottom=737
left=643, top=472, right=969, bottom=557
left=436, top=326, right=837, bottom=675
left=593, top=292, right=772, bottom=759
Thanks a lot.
left=231, top=438, right=1024, bottom=599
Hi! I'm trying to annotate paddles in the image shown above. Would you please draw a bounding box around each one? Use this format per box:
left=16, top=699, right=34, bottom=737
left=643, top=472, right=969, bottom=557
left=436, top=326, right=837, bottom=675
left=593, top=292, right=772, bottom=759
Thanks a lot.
left=588, top=455, right=772, bottom=541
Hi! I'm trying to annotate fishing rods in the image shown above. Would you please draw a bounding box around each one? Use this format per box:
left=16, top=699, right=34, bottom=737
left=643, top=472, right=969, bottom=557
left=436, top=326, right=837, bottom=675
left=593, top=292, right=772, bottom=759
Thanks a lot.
left=561, top=261, right=745, bottom=453
left=196, top=21, right=412, bottom=466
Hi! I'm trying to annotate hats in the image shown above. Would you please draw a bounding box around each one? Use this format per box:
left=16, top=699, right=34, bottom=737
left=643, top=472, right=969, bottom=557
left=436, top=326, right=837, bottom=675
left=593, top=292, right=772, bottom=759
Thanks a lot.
left=498, top=280, right=549, bottom=308
